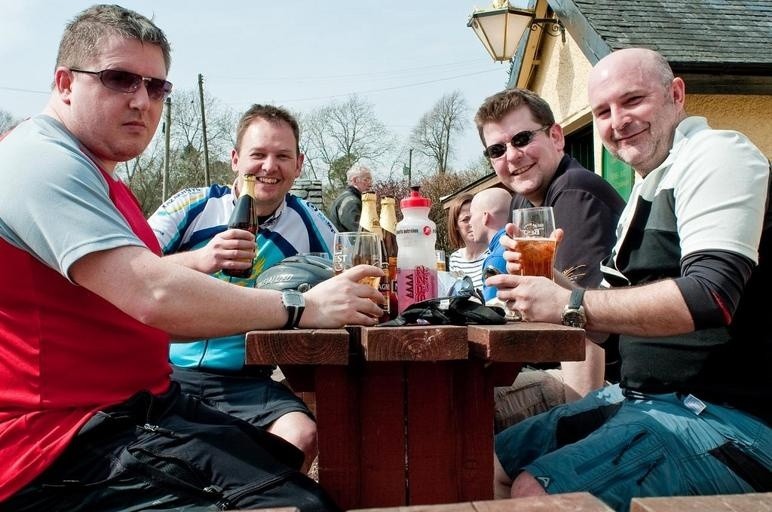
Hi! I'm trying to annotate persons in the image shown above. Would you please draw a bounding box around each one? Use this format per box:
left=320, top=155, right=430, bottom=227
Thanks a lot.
left=147, top=104, right=349, bottom=480
left=1, top=5, right=384, bottom=511
left=447, top=193, right=491, bottom=302
left=488, top=46, right=772, bottom=504
left=473, top=90, right=630, bottom=439
left=330, top=163, right=381, bottom=246
left=460, top=188, right=515, bottom=318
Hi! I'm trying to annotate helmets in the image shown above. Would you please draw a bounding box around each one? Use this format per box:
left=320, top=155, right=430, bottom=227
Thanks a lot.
left=255, top=254, right=335, bottom=292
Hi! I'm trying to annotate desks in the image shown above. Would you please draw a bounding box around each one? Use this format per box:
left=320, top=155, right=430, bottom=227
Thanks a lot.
left=238, top=321, right=590, bottom=508
left=218, top=493, right=772, bottom=512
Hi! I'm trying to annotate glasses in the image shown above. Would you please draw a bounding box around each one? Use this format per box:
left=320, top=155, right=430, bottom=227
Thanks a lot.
left=449, top=276, right=485, bottom=305
left=69, top=69, right=172, bottom=102
left=483, top=125, right=549, bottom=158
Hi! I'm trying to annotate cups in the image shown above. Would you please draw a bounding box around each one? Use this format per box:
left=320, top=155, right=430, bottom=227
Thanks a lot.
left=510, top=206, right=557, bottom=284
left=435, top=250, right=446, bottom=272
left=333, top=230, right=383, bottom=292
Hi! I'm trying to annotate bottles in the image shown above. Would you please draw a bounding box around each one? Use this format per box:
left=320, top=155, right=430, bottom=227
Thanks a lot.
left=352, top=191, right=390, bottom=324
left=219, top=173, right=260, bottom=280
left=395, top=185, right=439, bottom=316
left=379, top=194, right=398, bottom=317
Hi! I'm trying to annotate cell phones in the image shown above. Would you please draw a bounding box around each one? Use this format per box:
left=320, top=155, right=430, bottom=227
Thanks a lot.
left=483, top=265, right=503, bottom=279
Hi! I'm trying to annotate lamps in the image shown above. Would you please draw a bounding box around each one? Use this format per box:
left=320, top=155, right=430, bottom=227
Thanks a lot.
left=466, top=2, right=567, bottom=63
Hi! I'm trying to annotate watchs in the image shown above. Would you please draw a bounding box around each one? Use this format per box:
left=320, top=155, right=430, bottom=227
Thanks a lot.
left=282, top=288, right=305, bottom=329
left=561, top=288, right=588, bottom=329
